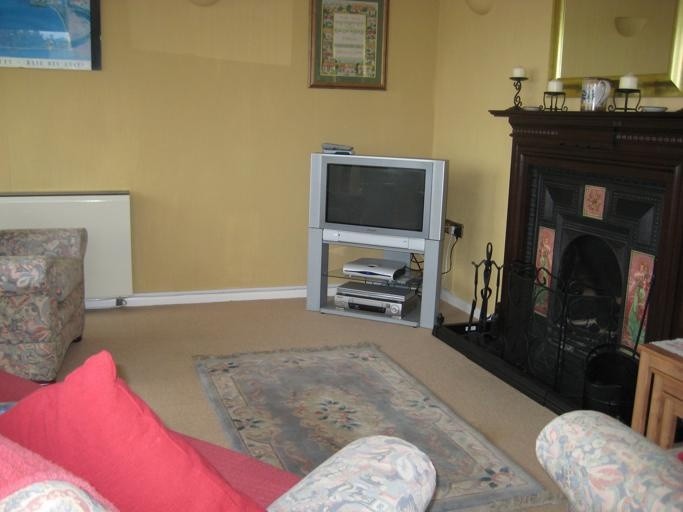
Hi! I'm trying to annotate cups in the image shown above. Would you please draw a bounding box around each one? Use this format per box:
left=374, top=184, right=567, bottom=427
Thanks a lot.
left=580, top=76, right=611, bottom=112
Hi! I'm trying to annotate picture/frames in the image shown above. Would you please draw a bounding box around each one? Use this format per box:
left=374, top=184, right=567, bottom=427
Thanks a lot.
left=307, top=0, right=390, bottom=91
left=547, top=0, right=683, bottom=97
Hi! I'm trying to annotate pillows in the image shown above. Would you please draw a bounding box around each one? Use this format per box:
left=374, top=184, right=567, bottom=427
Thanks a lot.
left=0, top=348, right=266, bottom=511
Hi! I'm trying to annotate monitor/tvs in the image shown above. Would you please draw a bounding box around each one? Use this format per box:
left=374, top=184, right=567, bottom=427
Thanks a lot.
left=307, top=151, right=448, bottom=241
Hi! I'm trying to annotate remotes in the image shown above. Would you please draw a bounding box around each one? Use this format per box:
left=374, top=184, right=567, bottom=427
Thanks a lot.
left=322, top=141, right=353, bottom=153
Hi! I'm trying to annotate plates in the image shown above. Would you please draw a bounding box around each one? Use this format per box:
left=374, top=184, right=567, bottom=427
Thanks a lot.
left=523, top=106, right=540, bottom=111
left=640, top=104, right=667, bottom=111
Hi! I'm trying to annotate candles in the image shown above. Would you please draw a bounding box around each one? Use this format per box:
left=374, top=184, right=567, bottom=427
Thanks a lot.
left=509, top=66, right=641, bottom=95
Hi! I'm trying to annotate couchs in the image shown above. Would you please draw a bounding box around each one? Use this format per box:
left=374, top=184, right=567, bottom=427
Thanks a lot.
left=0, top=429, right=438, bottom=511
left=533, top=407, right=683, bottom=512
left=0, top=228, right=93, bottom=382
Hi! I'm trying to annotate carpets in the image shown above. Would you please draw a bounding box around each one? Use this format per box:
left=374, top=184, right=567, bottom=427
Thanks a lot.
left=187, top=343, right=559, bottom=512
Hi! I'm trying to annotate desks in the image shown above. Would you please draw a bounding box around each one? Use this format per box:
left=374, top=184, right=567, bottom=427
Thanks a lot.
left=631, top=330, right=683, bottom=448
